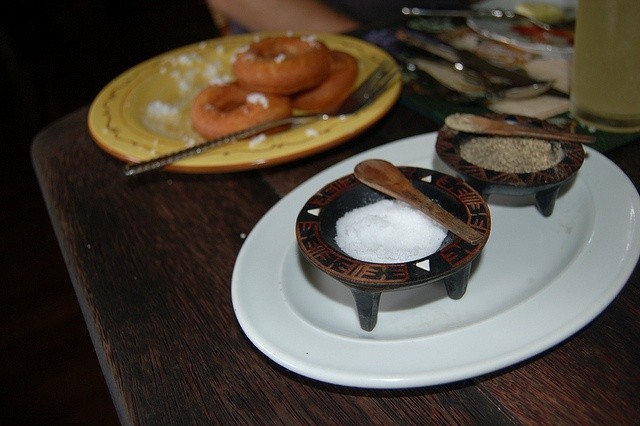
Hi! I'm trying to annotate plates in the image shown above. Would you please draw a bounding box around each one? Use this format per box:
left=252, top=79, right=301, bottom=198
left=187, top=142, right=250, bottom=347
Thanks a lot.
left=466, top=0, right=577, bottom=54
left=228, top=129, right=639, bottom=390
left=85, top=30, right=403, bottom=175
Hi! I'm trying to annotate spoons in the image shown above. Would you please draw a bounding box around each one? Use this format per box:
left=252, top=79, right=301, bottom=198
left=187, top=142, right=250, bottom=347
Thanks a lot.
left=445, top=113, right=596, bottom=143
left=354, top=159, right=483, bottom=246
left=395, top=27, right=557, bottom=98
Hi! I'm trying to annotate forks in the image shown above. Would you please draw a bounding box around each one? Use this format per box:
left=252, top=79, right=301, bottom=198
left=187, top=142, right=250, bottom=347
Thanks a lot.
left=121, top=57, right=403, bottom=176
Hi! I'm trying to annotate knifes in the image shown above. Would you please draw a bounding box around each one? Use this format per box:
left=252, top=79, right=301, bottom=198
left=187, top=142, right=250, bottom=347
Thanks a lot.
left=404, top=24, right=569, bottom=98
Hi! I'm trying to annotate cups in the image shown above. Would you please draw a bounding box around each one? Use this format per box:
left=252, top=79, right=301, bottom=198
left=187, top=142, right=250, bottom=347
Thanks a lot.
left=569, top=1, right=640, bottom=134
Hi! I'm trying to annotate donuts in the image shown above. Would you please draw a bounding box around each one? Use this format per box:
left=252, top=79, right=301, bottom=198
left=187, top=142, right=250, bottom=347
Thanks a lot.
left=230, top=36, right=331, bottom=95
left=189, top=84, right=294, bottom=141
left=290, top=51, right=358, bottom=114
